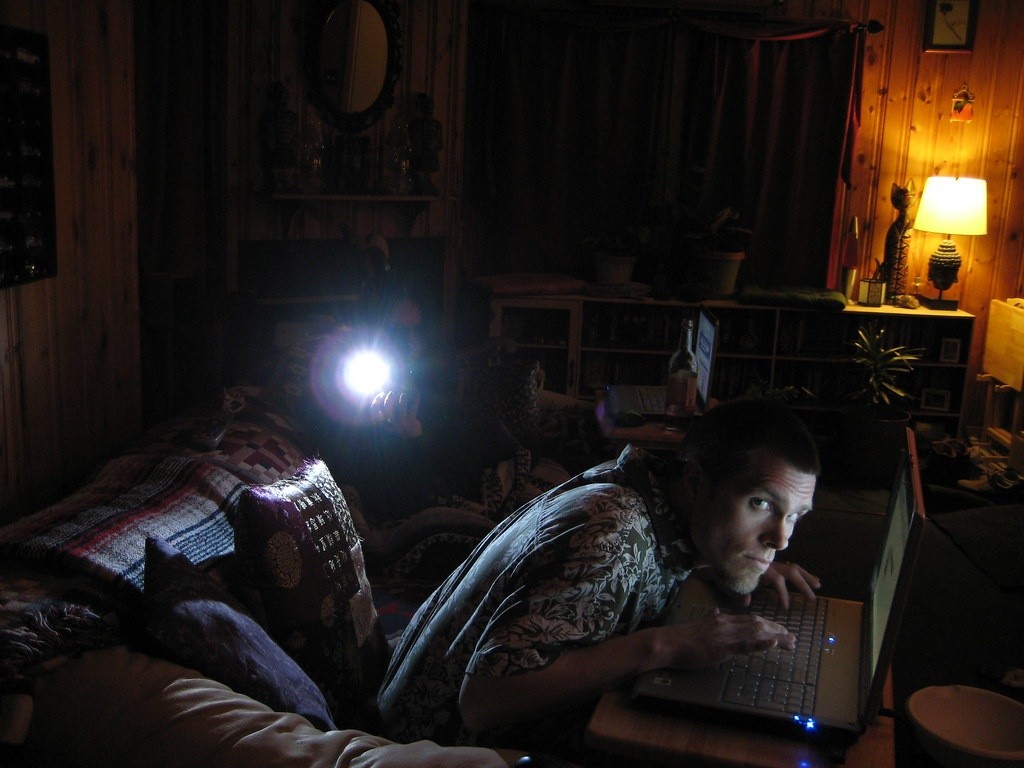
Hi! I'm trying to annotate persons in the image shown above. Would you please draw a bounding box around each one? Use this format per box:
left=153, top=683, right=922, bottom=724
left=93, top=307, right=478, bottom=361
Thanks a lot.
left=377, top=399, right=822, bottom=753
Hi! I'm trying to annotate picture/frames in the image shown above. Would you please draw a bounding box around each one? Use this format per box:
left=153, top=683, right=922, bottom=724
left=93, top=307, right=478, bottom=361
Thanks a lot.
left=920, top=387, right=952, bottom=413
left=939, top=336, right=961, bottom=365
left=923, top=0, right=980, bottom=54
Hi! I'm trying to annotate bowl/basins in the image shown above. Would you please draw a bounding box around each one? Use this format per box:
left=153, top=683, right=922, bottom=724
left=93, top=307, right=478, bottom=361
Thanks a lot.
left=905, top=685, right=1024, bottom=768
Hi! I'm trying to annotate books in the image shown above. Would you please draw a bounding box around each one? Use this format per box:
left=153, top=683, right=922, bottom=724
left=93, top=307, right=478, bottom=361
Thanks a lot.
left=578, top=352, right=952, bottom=409
left=503, top=303, right=938, bottom=363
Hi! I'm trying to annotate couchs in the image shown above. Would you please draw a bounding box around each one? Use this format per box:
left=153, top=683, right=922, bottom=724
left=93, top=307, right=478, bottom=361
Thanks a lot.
left=0, top=357, right=546, bottom=768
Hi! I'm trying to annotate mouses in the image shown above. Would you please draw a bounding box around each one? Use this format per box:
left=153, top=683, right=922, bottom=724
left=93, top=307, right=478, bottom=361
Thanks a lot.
left=612, top=410, right=646, bottom=427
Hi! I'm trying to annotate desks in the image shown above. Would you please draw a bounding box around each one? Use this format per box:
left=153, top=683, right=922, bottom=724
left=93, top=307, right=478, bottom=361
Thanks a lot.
left=583, top=575, right=899, bottom=768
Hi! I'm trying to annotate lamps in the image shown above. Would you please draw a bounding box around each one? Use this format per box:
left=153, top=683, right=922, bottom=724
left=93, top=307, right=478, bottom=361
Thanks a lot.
left=912, top=176, right=989, bottom=311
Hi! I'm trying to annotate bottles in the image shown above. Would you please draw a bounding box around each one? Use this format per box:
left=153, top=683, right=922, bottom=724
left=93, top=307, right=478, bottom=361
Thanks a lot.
left=664, top=318, right=698, bottom=433
left=301, top=111, right=326, bottom=194
left=391, top=116, right=414, bottom=195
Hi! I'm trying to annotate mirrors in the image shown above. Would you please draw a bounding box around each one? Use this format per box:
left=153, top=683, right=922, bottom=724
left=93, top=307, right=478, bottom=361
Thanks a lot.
left=303, top=0, right=407, bottom=136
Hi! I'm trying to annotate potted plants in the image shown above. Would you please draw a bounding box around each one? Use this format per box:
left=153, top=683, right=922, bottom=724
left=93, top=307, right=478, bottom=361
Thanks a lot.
left=837, top=316, right=929, bottom=479
left=574, top=216, right=654, bottom=293
left=670, top=199, right=748, bottom=297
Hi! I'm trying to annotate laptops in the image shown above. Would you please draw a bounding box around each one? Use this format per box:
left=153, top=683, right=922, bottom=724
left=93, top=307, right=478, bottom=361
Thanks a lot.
left=631, top=427, right=927, bottom=742
left=604, top=303, right=720, bottom=418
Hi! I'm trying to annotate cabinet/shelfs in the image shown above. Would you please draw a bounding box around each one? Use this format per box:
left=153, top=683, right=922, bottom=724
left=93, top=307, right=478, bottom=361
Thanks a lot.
left=484, top=288, right=978, bottom=462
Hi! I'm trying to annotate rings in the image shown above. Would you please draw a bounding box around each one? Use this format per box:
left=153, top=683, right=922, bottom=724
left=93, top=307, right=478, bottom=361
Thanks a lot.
left=781, top=561, right=791, bottom=574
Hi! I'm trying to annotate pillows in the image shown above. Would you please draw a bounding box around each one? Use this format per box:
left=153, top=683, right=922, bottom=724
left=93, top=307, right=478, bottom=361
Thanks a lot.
left=234, top=451, right=387, bottom=700
left=138, top=533, right=337, bottom=732
